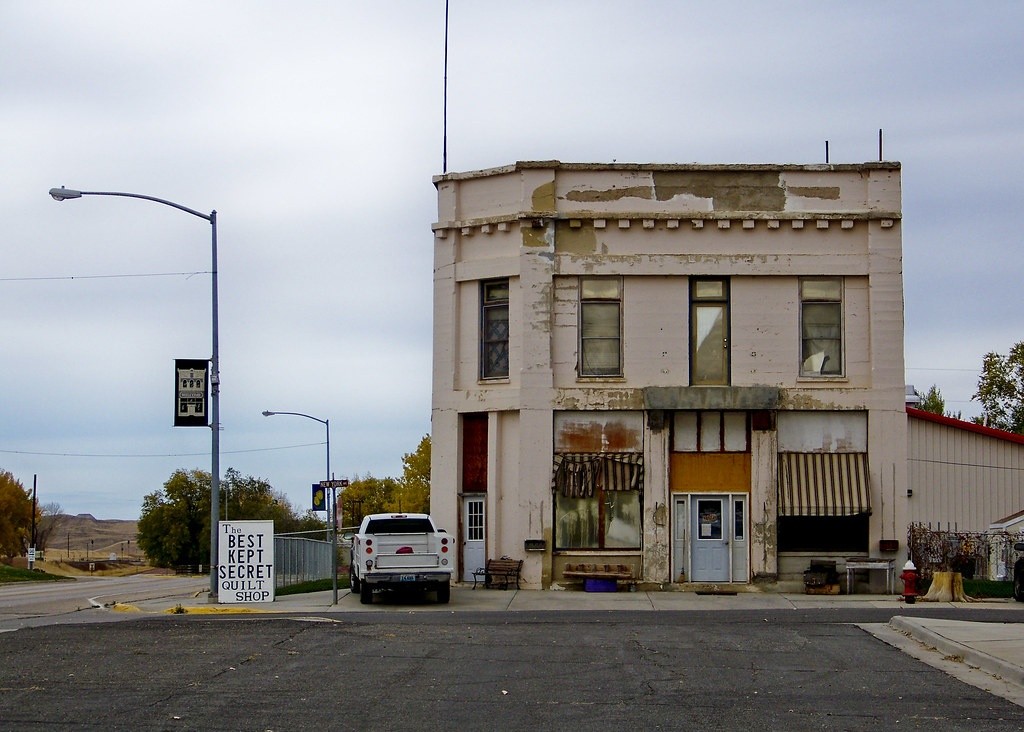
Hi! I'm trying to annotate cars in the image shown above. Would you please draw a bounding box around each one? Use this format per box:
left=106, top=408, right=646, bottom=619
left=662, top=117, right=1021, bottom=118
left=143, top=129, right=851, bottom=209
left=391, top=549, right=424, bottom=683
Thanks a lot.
left=1013, top=542, right=1024, bottom=602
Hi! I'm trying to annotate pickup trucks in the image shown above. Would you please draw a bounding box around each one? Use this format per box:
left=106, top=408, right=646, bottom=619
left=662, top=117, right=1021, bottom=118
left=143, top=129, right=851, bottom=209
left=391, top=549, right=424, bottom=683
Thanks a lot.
left=344, top=512, right=452, bottom=604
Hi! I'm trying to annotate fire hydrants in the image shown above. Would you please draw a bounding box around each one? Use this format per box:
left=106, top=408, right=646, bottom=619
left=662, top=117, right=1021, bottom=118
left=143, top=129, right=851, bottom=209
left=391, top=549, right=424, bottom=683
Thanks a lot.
left=899, top=561, right=918, bottom=604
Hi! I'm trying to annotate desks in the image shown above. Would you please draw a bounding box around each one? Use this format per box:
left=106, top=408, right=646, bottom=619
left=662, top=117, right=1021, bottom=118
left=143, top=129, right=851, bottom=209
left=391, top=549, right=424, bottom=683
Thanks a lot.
left=846, top=558, right=896, bottom=595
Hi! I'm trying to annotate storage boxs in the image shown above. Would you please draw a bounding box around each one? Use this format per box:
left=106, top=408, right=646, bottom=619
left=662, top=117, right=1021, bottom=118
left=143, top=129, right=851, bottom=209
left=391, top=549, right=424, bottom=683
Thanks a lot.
left=583, top=578, right=617, bottom=592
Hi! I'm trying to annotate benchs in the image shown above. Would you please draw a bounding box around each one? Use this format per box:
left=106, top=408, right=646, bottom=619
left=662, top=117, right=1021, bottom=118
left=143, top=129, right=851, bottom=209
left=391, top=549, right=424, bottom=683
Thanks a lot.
left=472, top=559, right=524, bottom=590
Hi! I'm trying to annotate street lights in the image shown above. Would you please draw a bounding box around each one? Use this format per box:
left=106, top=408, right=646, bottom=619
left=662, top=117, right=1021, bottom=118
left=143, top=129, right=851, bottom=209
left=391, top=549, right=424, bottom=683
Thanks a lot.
left=49, top=186, right=220, bottom=598
left=262, top=410, right=330, bottom=542
